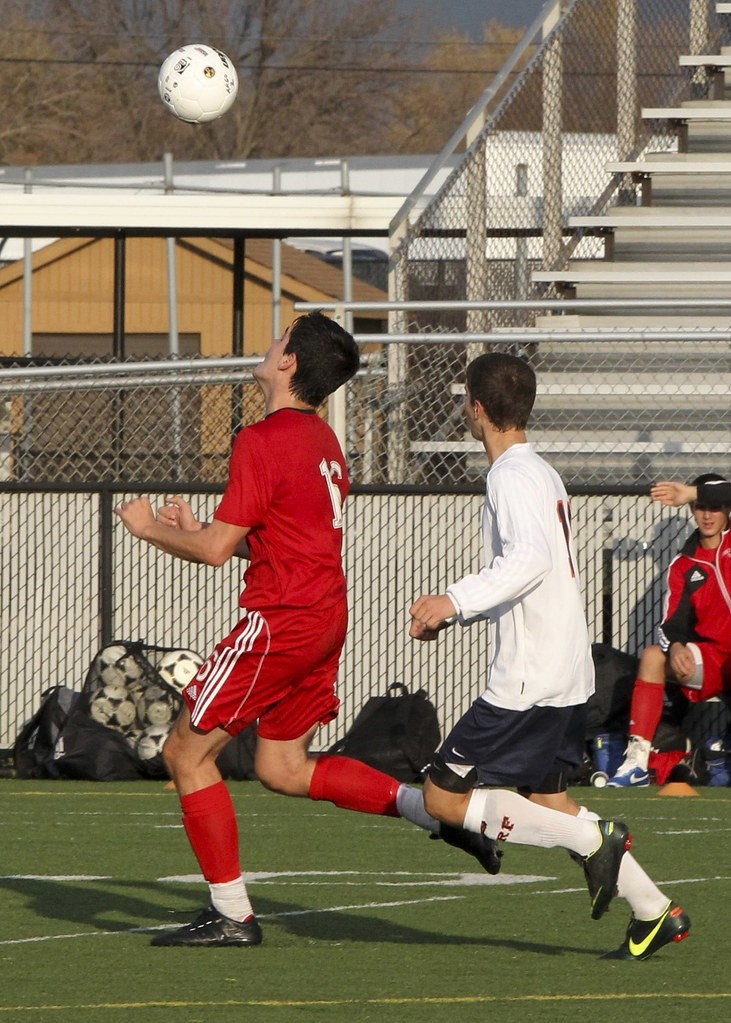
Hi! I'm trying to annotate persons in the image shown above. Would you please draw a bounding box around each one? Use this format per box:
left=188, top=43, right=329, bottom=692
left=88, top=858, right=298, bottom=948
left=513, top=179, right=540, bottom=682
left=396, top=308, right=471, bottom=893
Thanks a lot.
left=606, top=475, right=731, bottom=790
left=115, top=310, right=505, bottom=946
left=409, top=353, right=692, bottom=960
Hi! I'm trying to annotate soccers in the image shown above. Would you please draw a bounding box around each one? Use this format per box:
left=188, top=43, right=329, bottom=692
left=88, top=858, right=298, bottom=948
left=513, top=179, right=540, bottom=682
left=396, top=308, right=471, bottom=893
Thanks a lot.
left=158, top=44, right=241, bottom=124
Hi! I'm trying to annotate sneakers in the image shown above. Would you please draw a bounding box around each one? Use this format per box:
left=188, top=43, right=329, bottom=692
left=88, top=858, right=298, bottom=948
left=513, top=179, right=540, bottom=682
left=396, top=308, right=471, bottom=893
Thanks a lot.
left=600, top=899, right=692, bottom=961
left=568, top=819, right=633, bottom=920
left=606, top=758, right=650, bottom=788
left=429, top=821, right=504, bottom=875
left=151, top=903, right=263, bottom=948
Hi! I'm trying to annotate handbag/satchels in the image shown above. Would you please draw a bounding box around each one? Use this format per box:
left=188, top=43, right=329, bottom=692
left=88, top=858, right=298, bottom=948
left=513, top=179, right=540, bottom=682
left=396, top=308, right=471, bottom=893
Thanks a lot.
left=13, top=640, right=260, bottom=781
left=567, top=643, right=731, bottom=788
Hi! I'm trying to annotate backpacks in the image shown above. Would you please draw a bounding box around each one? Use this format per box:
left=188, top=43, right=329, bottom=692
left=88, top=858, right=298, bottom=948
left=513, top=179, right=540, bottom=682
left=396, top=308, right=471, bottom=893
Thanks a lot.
left=326, top=682, right=442, bottom=785
